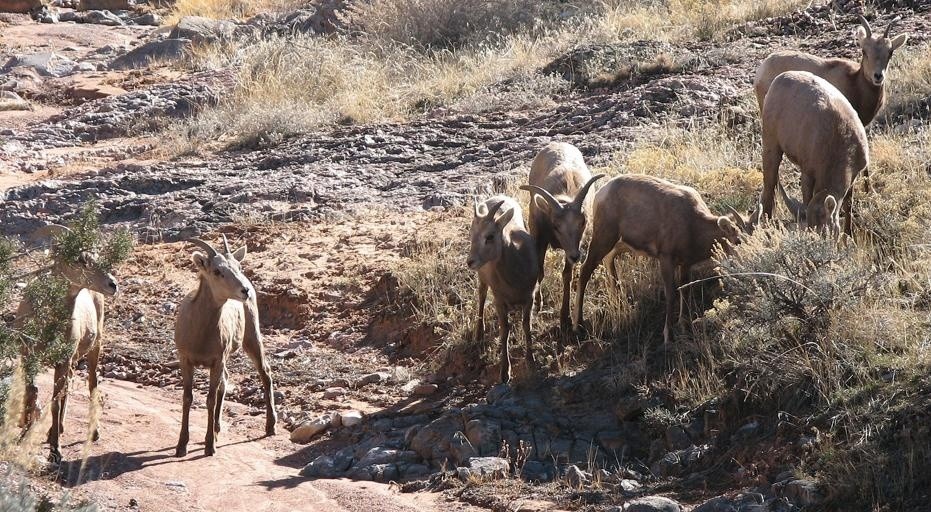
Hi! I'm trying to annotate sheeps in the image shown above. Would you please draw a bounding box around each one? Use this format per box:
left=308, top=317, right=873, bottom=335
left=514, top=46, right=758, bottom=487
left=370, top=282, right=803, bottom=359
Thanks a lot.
left=576, top=172, right=764, bottom=342
left=759, top=68, right=871, bottom=246
left=519, top=141, right=607, bottom=336
left=171, top=230, right=277, bottom=458
left=16, top=221, right=118, bottom=463
left=750, top=10, right=908, bottom=130
left=464, top=192, right=540, bottom=383
